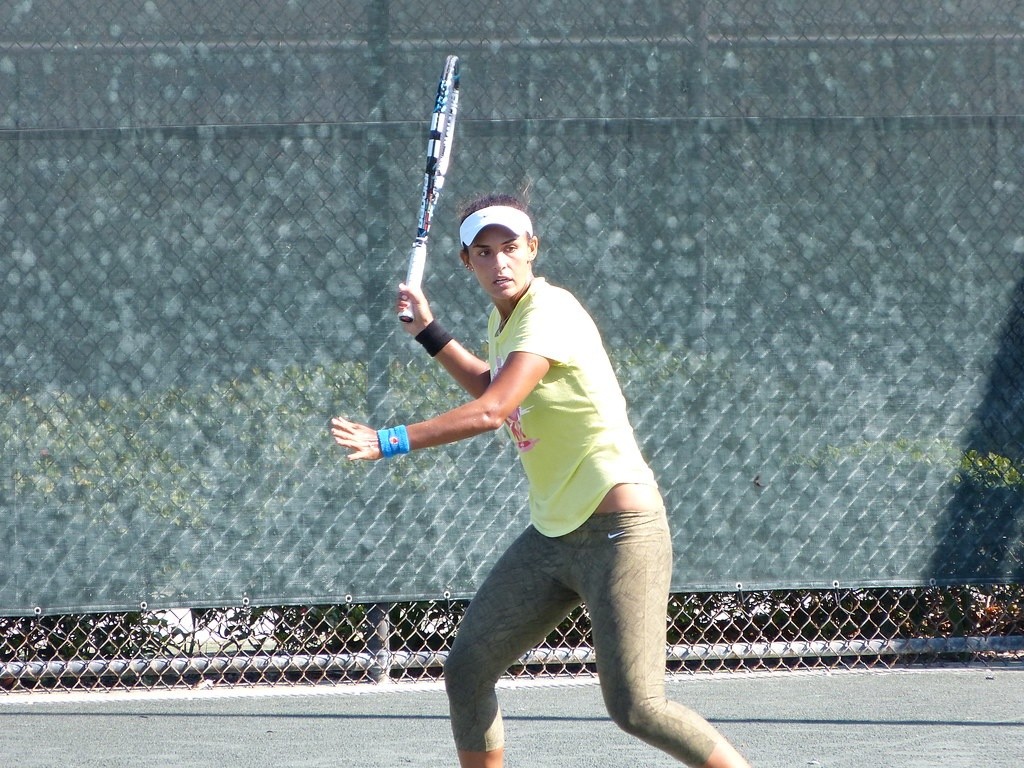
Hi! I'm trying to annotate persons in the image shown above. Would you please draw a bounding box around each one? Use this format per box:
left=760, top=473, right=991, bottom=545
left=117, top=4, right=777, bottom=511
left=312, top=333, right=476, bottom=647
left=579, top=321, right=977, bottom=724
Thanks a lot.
left=330, top=193, right=754, bottom=768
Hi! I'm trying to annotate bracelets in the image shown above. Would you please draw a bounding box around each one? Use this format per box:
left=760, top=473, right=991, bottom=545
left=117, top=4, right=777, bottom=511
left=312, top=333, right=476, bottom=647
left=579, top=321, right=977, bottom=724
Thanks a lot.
left=377, top=424, right=411, bottom=458
left=414, top=320, right=453, bottom=358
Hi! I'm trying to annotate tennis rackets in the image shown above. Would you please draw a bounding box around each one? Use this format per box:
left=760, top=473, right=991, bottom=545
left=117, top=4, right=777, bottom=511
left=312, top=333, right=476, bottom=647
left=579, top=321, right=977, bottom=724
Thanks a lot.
left=396, top=55, right=461, bottom=323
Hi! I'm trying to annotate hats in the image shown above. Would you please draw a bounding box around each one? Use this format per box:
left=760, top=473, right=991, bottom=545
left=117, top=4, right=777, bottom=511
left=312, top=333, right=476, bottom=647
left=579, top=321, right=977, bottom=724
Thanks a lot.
left=460, top=204, right=533, bottom=247
